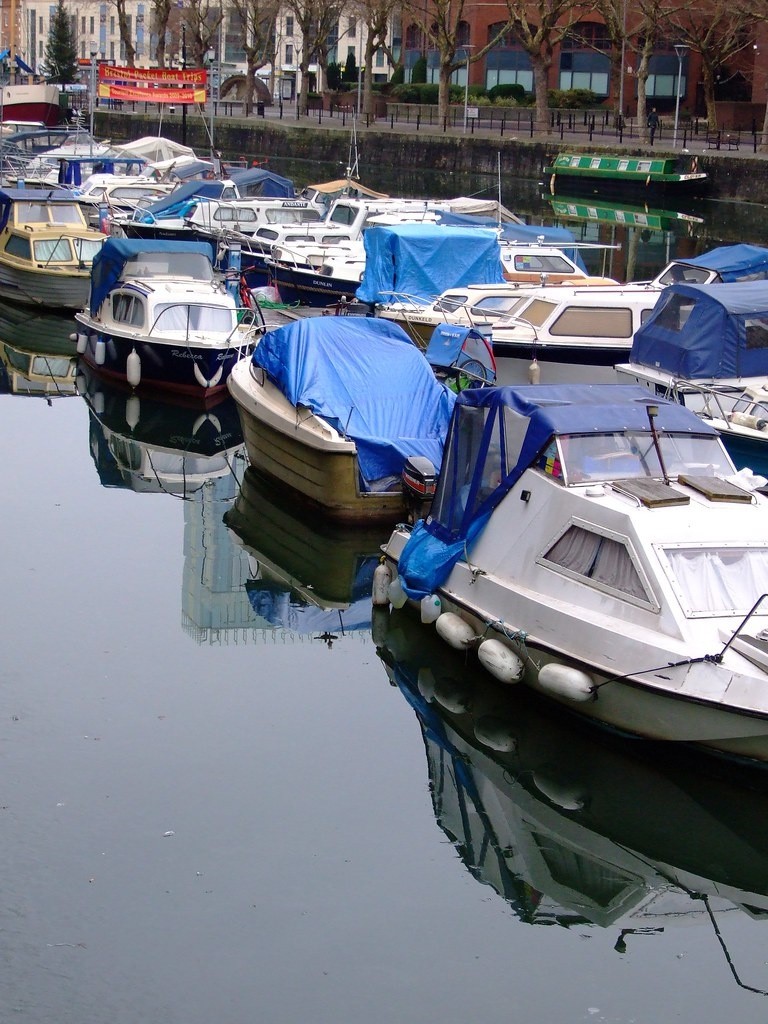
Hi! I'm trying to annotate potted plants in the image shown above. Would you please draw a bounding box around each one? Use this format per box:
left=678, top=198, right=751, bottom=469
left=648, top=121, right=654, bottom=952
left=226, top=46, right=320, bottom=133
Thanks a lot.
left=298, top=92, right=322, bottom=110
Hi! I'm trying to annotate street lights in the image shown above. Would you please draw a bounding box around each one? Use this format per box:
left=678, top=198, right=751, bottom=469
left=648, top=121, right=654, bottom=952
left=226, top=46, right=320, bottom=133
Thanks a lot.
left=672, top=43, right=691, bottom=149
left=461, top=45, right=476, bottom=134
left=293, top=41, right=302, bottom=119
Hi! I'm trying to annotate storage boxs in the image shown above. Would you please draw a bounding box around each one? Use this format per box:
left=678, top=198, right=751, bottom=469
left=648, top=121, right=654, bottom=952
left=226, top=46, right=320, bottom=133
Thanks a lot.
left=582, top=455, right=641, bottom=476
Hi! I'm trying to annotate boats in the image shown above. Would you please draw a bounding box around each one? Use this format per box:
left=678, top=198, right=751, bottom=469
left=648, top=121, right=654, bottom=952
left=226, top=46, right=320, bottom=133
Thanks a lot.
left=0, top=82, right=589, bottom=313
left=0, top=296, right=81, bottom=408
left=370, top=642, right=768, bottom=954
left=70, top=356, right=244, bottom=494
left=539, top=191, right=710, bottom=232
left=379, top=248, right=651, bottom=349
left=430, top=240, right=768, bottom=368
left=612, top=277, right=768, bottom=420
left=670, top=378, right=768, bottom=500
left=226, top=316, right=481, bottom=515
left=372, top=385, right=768, bottom=757
left=69, top=236, right=283, bottom=409
left=544, top=151, right=709, bottom=197
left=218, top=467, right=380, bottom=651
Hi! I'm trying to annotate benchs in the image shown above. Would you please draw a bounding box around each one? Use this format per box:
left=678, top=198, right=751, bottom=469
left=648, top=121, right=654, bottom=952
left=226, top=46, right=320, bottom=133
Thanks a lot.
left=213, top=99, right=265, bottom=117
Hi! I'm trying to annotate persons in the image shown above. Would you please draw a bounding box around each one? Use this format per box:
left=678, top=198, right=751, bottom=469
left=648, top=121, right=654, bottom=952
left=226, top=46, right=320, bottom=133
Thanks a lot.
left=647, top=107, right=659, bottom=143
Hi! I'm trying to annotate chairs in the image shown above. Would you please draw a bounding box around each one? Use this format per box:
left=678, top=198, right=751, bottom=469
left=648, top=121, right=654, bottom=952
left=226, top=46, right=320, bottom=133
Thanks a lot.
left=708, top=129, right=721, bottom=149
left=728, top=136, right=740, bottom=150
